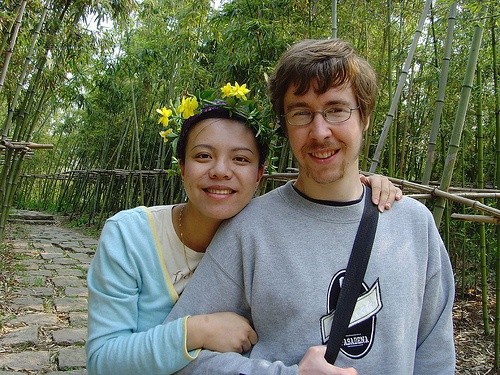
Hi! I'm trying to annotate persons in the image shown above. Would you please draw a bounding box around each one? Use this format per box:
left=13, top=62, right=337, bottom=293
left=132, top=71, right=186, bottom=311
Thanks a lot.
left=83, top=104, right=403, bottom=375
left=158, top=37, right=458, bottom=375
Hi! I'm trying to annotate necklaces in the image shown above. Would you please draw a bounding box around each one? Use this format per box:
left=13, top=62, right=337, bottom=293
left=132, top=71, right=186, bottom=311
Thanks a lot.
left=178, top=203, right=197, bottom=273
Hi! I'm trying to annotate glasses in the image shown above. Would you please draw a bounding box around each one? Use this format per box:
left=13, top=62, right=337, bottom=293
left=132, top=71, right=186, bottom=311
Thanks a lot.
left=279, top=105, right=361, bottom=128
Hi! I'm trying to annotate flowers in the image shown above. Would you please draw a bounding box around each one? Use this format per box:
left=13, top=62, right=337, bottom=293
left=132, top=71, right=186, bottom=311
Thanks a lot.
left=156, top=81, right=277, bottom=179
